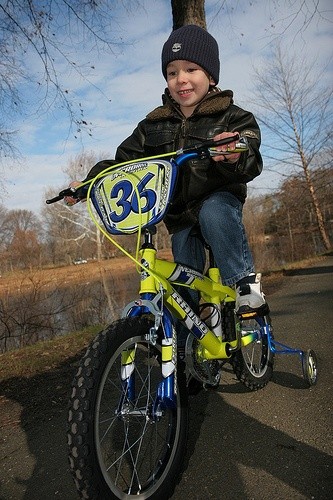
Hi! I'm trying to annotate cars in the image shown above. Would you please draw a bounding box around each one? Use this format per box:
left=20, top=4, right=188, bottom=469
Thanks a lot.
left=73, top=257, right=88, bottom=265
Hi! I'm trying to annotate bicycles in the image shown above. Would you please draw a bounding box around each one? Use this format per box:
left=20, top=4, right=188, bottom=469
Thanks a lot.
left=42, top=132, right=320, bottom=500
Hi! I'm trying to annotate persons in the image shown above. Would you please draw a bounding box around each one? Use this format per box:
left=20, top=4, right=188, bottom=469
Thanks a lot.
left=62, top=25, right=265, bottom=368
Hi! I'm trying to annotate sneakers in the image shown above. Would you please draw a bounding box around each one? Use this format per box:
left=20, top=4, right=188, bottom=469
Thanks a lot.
left=231, top=272, right=269, bottom=317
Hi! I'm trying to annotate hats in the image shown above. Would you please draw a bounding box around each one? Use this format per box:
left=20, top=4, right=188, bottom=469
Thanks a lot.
left=161, top=24, right=219, bottom=87
